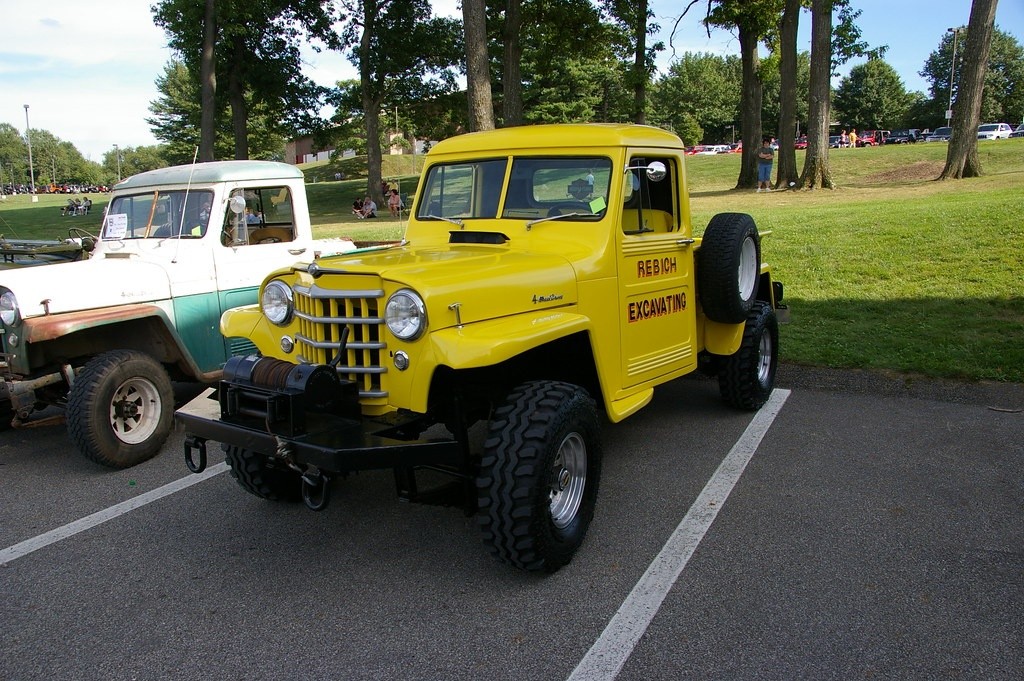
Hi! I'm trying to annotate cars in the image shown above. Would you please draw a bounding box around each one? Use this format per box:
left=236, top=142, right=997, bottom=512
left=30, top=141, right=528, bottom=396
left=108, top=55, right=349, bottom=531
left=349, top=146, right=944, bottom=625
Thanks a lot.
left=769, top=139, right=778, bottom=149
left=795, top=136, right=807, bottom=149
left=0, top=184, right=112, bottom=194
left=685, top=141, right=742, bottom=155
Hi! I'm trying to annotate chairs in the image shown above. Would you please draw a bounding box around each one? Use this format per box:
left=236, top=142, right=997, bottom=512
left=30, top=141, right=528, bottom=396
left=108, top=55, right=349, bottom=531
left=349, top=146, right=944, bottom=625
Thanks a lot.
left=69, top=200, right=92, bottom=216
left=396, top=193, right=408, bottom=216
left=248, top=227, right=292, bottom=246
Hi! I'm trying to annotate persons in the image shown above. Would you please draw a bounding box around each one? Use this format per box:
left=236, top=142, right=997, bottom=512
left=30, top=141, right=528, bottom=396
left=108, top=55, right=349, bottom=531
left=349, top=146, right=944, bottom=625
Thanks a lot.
left=335, top=172, right=344, bottom=179
left=245, top=209, right=266, bottom=223
left=200, top=203, right=211, bottom=220
left=848, top=129, right=856, bottom=148
left=586, top=169, right=594, bottom=199
left=62, top=197, right=90, bottom=217
left=64, top=183, right=68, bottom=194
left=757, top=138, right=774, bottom=193
left=839, top=130, right=846, bottom=147
left=352, top=178, right=405, bottom=220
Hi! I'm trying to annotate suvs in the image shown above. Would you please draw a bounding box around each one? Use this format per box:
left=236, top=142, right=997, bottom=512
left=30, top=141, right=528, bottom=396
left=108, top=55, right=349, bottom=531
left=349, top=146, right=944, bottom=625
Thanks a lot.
left=176, top=124, right=788, bottom=577
left=978, top=123, right=1024, bottom=140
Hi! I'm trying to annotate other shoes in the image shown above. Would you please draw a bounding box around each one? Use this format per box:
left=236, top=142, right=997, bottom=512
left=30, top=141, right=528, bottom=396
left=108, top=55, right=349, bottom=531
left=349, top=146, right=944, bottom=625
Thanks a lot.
left=765, top=188, right=771, bottom=192
left=757, top=188, right=762, bottom=193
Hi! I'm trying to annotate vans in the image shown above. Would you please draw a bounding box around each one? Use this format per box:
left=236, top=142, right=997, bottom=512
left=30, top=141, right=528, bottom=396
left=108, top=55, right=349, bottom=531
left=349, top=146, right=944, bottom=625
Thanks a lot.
left=829, top=127, right=951, bottom=148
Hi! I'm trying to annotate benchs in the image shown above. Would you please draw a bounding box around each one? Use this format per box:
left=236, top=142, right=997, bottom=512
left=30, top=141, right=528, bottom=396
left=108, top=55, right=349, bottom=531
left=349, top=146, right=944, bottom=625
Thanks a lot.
left=1, top=246, right=80, bottom=263
left=504, top=208, right=674, bottom=234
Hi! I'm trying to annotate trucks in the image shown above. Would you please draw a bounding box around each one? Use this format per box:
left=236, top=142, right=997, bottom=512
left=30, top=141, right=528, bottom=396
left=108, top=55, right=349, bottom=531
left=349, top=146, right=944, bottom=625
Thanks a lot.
left=0, top=161, right=404, bottom=469
